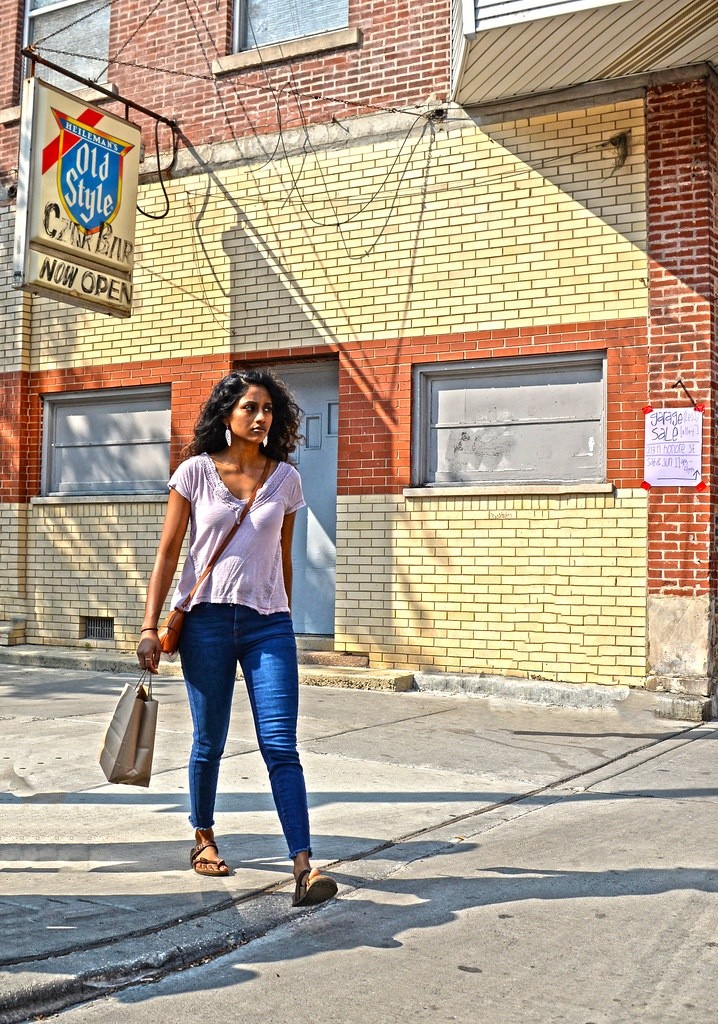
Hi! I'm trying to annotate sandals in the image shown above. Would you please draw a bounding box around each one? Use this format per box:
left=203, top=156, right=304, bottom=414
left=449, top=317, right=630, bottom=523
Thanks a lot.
left=292, top=869, right=338, bottom=908
left=190, top=842, right=229, bottom=875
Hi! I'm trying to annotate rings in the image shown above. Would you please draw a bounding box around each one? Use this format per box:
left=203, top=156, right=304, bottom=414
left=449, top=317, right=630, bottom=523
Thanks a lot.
left=145, top=658, right=152, bottom=661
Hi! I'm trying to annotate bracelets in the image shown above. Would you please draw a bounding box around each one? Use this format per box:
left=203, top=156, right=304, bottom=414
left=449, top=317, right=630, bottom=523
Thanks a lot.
left=140, top=627, right=157, bottom=633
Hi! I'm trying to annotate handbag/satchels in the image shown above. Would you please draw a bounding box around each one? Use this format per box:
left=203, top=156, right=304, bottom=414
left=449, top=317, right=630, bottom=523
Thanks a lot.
left=99, top=668, right=159, bottom=788
left=157, top=605, right=186, bottom=663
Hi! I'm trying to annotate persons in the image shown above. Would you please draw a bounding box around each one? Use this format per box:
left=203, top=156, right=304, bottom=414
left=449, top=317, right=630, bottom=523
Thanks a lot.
left=137, top=370, right=338, bottom=908
